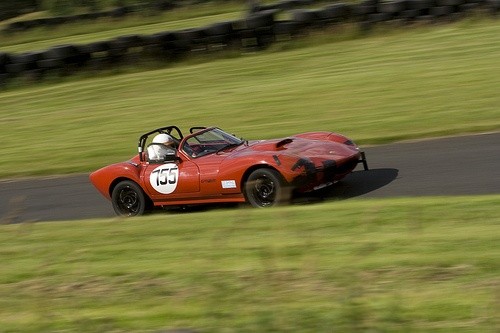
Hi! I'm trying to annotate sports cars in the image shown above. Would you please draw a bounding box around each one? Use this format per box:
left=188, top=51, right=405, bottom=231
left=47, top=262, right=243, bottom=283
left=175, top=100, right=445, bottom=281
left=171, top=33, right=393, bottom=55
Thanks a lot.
left=88, top=125, right=369, bottom=220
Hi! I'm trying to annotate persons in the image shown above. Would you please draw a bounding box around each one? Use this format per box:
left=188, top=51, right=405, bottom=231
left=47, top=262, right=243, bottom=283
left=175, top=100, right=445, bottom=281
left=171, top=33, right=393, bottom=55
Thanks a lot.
left=146, top=134, right=179, bottom=160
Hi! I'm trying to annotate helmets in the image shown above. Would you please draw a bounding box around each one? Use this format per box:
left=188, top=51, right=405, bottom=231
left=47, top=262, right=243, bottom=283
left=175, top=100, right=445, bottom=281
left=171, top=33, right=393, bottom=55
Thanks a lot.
left=153, top=134, right=176, bottom=157
